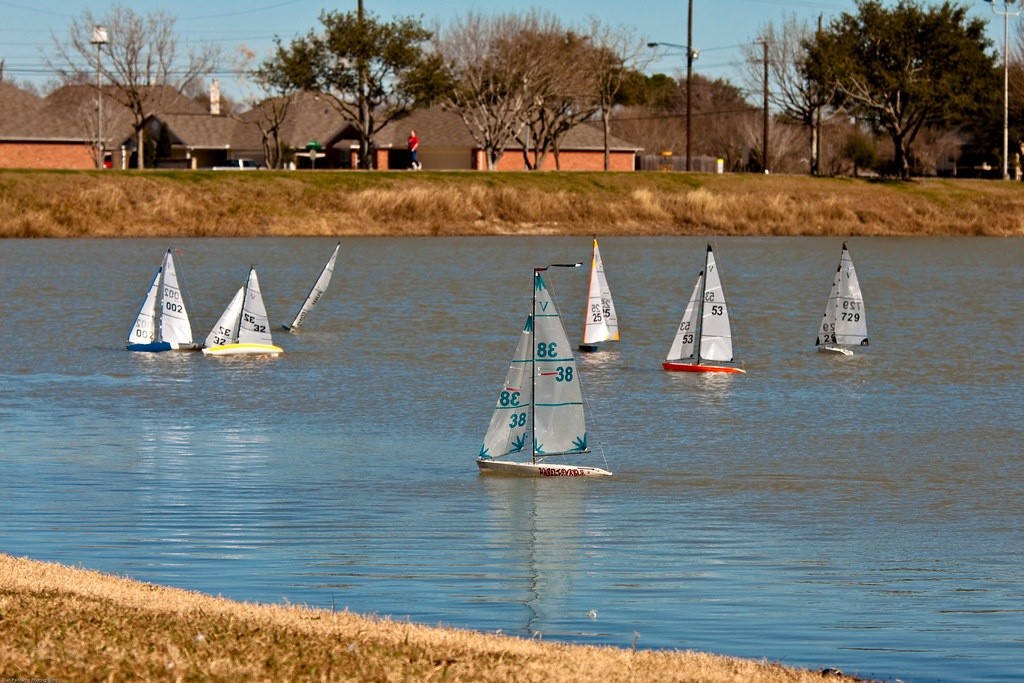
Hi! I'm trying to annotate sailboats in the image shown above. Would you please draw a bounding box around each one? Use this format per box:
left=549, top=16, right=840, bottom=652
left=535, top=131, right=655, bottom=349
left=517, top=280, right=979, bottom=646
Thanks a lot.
left=475, top=261, right=615, bottom=479
left=663, top=242, right=749, bottom=375
left=577, top=232, right=620, bottom=353
left=124, top=244, right=202, bottom=354
left=281, top=239, right=342, bottom=335
left=201, top=267, right=284, bottom=356
left=814, top=240, right=870, bottom=357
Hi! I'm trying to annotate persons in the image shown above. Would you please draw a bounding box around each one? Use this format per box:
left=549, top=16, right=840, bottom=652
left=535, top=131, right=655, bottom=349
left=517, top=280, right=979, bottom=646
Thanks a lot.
left=408, top=129, right=422, bottom=171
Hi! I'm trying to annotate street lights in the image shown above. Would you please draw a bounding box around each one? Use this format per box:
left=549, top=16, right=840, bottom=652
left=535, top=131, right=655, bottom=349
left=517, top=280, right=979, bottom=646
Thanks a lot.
left=984, top=0, right=1024, bottom=178
left=90, top=25, right=110, bottom=171
left=645, top=40, right=694, bottom=172
